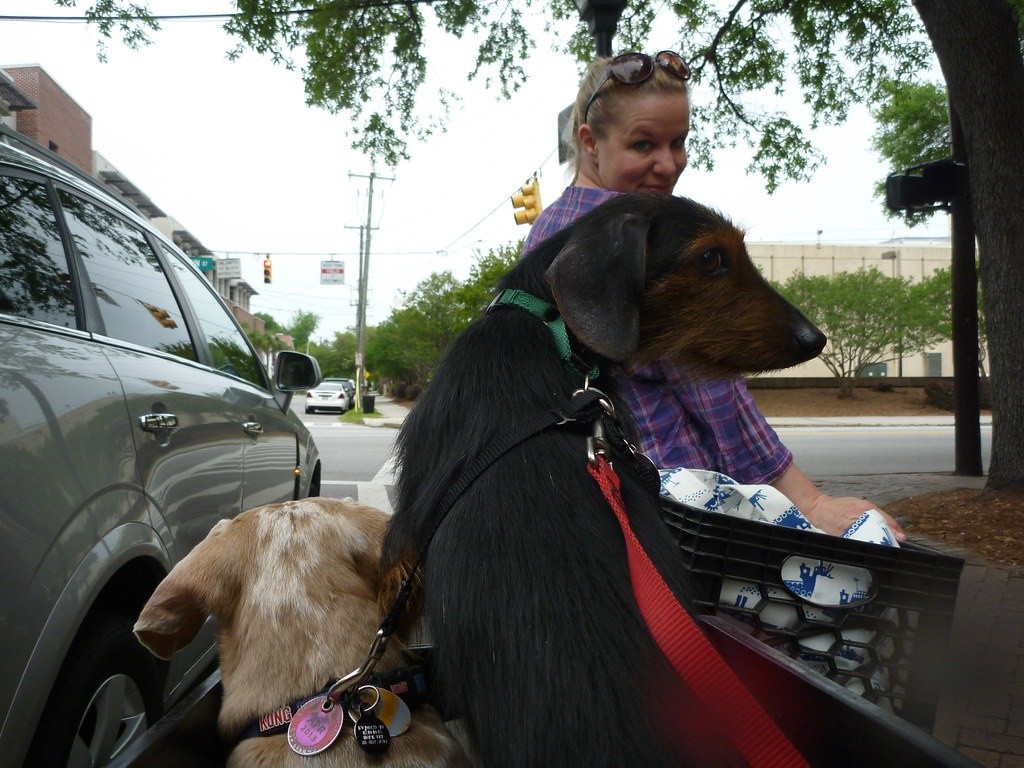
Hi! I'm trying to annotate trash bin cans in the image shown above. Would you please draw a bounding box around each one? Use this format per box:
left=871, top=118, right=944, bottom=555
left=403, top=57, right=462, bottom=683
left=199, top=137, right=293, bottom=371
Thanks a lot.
left=362, top=395, right=375, bottom=414
left=362, top=386, right=368, bottom=396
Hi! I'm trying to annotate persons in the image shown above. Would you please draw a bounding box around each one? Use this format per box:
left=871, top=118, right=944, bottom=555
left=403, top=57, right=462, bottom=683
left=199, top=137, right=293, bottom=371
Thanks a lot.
left=519, top=51, right=909, bottom=542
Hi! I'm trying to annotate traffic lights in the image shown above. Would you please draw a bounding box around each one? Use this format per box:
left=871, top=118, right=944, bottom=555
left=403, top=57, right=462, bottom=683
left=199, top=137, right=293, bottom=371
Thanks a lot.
left=264, top=260, right=272, bottom=284
left=511, top=178, right=543, bottom=225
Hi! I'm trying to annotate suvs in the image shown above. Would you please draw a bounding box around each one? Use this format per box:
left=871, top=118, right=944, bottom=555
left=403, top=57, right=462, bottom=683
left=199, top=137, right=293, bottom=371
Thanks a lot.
left=0, top=121, right=321, bottom=768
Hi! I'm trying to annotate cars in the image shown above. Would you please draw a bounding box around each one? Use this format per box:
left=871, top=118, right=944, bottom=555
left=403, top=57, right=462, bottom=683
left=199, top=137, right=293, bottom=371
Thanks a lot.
left=305, top=379, right=356, bottom=414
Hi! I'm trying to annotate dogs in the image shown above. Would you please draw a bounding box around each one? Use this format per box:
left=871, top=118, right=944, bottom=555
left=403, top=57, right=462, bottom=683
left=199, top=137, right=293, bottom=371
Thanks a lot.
left=133, top=191, right=827, bottom=768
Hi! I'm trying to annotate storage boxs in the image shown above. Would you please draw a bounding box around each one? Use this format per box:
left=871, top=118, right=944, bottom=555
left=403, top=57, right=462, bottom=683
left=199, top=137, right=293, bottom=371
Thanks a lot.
left=659, top=494, right=966, bottom=735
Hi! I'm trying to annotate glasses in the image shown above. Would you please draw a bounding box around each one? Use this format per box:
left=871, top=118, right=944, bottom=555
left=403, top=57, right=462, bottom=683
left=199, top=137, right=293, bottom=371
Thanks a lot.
left=584, top=50, right=691, bottom=125
